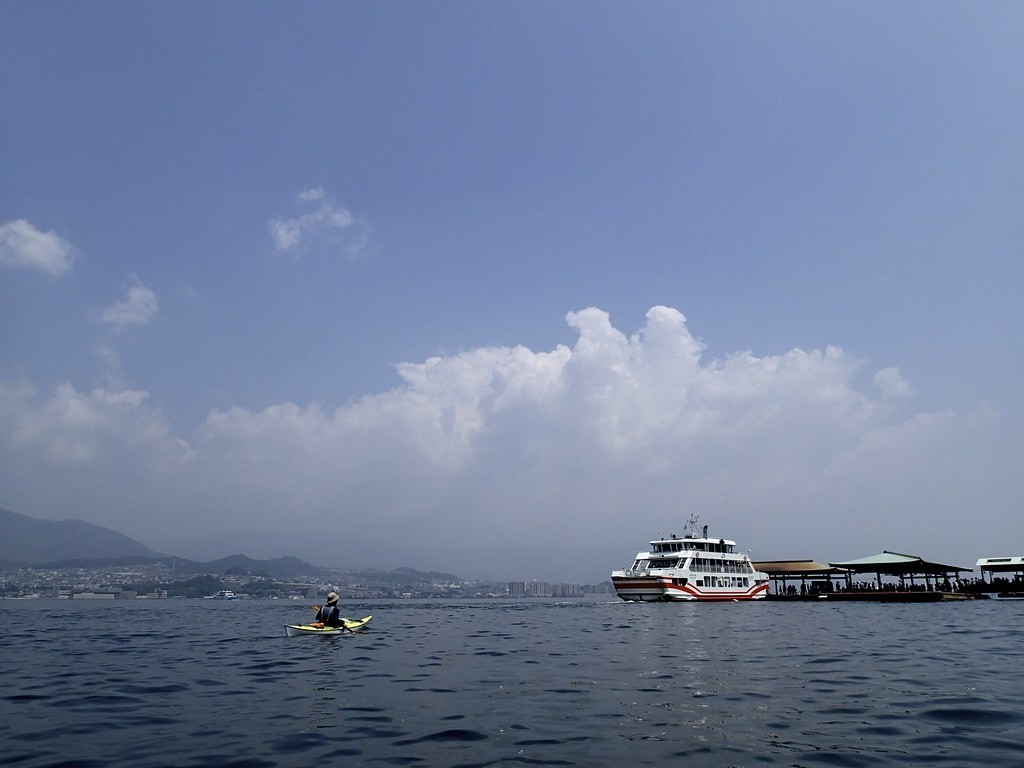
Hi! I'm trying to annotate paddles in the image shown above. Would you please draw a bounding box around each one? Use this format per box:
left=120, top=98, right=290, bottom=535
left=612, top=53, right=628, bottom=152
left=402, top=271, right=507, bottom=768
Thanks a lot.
left=311, top=605, right=356, bottom=634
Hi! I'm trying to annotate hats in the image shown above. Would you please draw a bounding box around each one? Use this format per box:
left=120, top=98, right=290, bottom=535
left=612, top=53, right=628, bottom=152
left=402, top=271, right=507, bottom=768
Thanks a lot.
left=326, top=592, right=339, bottom=603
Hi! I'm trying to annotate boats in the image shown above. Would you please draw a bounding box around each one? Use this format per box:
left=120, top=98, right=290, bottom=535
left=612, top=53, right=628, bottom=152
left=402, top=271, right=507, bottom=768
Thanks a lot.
left=611, top=513, right=770, bottom=603
left=213, top=589, right=237, bottom=600
left=284, top=615, right=373, bottom=637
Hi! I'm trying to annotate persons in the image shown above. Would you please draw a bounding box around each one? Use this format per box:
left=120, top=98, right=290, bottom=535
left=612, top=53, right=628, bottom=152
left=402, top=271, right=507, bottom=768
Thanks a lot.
left=779, top=577, right=1015, bottom=596
left=315, top=592, right=345, bottom=626
